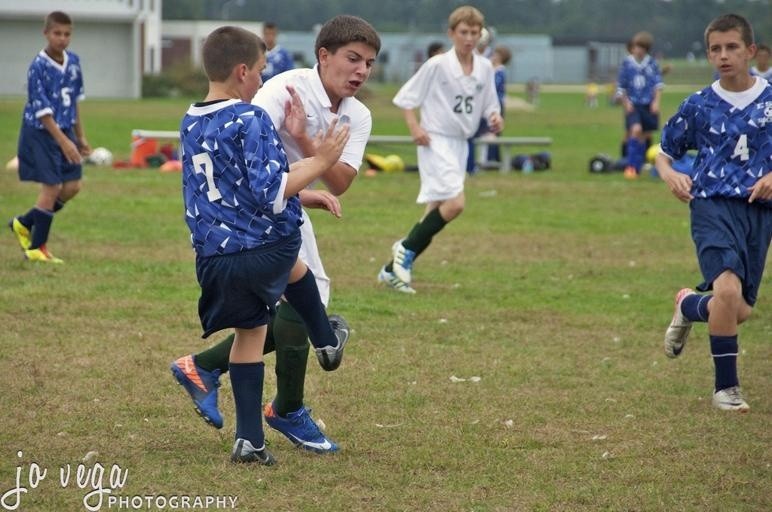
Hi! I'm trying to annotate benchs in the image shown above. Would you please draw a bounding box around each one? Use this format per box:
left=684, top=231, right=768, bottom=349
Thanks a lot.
left=129, top=127, right=553, bottom=172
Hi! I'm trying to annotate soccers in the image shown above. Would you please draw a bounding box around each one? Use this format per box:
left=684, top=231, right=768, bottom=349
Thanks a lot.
left=86, top=146, right=113, bottom=167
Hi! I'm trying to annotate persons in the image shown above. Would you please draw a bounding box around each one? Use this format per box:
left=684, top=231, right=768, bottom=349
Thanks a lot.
left=654, top=14, right=771, bottom=416
left=179, top=26, right=350, bottom=467
left=618, top=34, right=660, bottom=178
left=489, top=42, right=511, bottom=169
left=168, top=17, right=378, bottom=455
left=623, top=39, right=671, bottom=157
left=750, top=46, right=771, bottom=80
left=378, top=4, right=505, bottom=297
left=427, top=43, right=447, bottom=56
left=259, top=22, right=293, bottom=80
left=9, top=10, right=91, bottom=263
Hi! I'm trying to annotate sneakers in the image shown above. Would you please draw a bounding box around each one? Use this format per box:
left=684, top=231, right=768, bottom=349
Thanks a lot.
left=9, top=215, right=32, bottom=251
left=169, top=354, right=223, bottom=429
left=314, top=315, right=349, bottom=370
left=393, top=239, right=414, bottom=283
left=378, top=266, right=415, bottom=295
left=231, top=438, right=277, bottom=466
left=265, top=401, right=337, bottom=453
left=664, top=288, right=697, bottom=358
left=712, top=386, right=749, bottom=413
left=25, top=244, right=64, bottom=264
